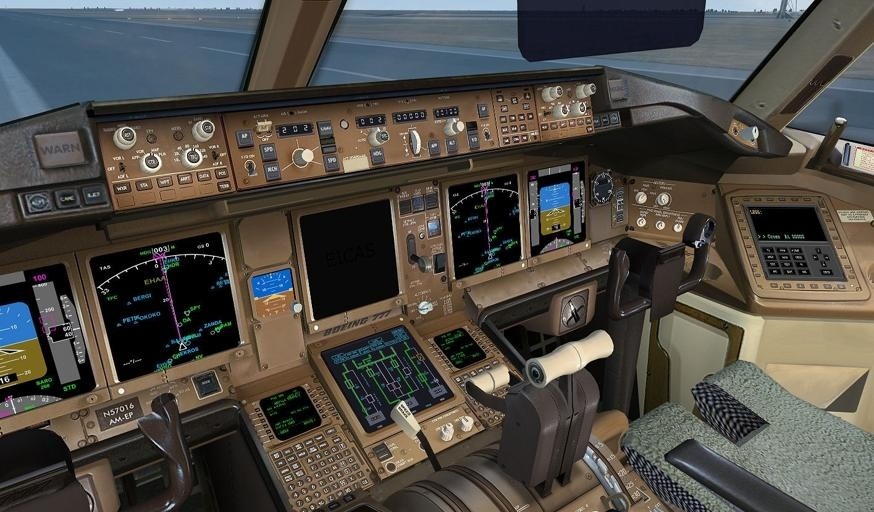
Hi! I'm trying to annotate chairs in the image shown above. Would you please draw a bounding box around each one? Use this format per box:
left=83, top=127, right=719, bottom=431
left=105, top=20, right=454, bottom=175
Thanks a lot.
left=620, top=362, right=873, bottom=511
